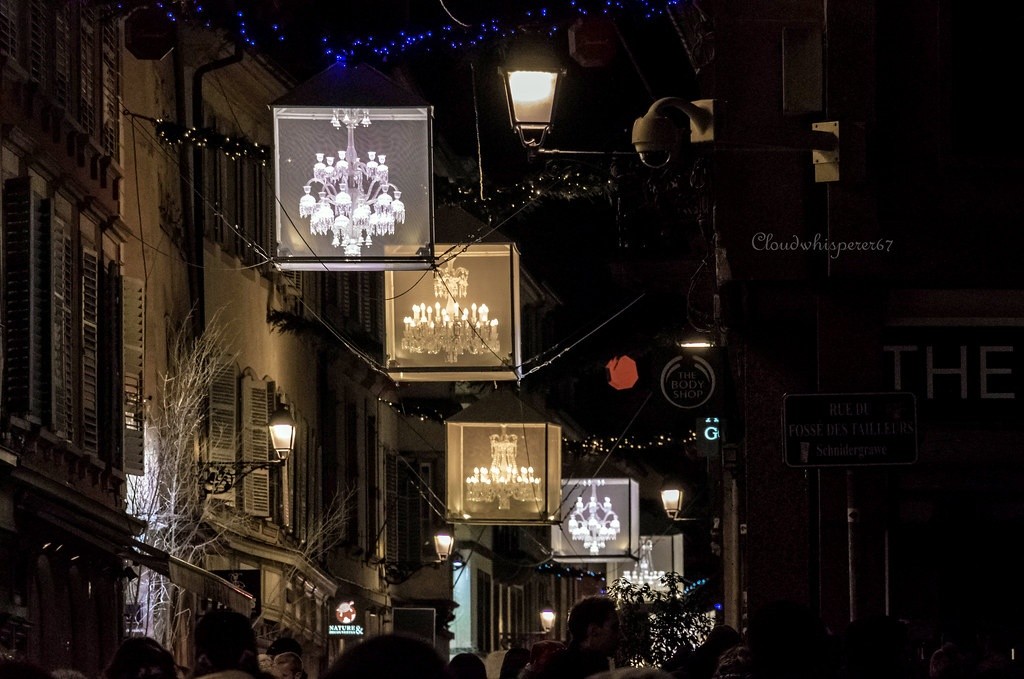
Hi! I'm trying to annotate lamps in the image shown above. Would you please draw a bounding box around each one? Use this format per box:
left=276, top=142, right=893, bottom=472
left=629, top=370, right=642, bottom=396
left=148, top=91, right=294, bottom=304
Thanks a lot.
left=443, top=390, right=561, bottom=527
left=199, top=392, right=297, bottom=495
left=381, top=528, right=455, bottom=589
left=266, top=56, right=435, bottom=274
left=550, top=460, right=640, bottom=563
left=384, top=243, right=524, bottom=387
left=496, top=32, right=635, bottom=158
left=604, top=509, right=685, bottom=604
left=658, top=477, right=701, bottom=523
left=499, top=606, right=558, bottom=649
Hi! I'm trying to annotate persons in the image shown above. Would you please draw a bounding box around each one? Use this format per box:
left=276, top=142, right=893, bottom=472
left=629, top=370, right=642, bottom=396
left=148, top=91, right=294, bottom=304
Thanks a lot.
left=0, top=597, right=1024, bottom=678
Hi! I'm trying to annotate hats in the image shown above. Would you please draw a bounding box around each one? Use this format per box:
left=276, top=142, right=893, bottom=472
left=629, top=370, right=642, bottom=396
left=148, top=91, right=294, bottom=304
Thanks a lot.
left=529, top=640, right=567, bottom=673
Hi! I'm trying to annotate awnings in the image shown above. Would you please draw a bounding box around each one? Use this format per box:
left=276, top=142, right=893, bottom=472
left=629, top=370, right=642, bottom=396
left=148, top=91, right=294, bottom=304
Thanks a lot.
left=11, top=488, right=254, bottom=617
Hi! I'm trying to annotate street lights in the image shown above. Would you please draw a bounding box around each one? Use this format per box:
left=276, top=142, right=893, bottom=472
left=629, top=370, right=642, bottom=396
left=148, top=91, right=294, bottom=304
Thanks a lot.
left=498, top=40, right=742, bottom=634
